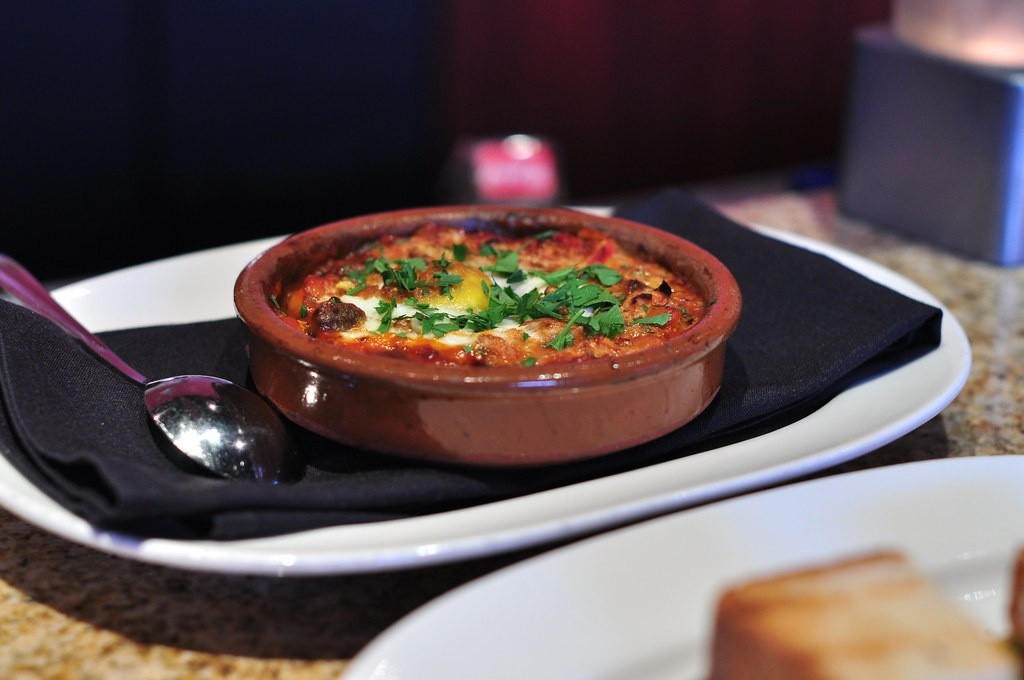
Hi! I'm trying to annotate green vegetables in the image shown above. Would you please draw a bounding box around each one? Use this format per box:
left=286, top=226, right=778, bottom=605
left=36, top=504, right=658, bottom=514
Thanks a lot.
left=268, top=226, right=674, bottom=364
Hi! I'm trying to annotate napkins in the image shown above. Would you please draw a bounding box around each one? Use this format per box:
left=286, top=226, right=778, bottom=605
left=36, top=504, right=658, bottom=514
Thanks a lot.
left=0, top=187, right=945, bottom=542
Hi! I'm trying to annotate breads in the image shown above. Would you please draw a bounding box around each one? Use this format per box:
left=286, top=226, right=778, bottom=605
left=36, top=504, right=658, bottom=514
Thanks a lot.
left=708, top=550, right=1024, bottom=680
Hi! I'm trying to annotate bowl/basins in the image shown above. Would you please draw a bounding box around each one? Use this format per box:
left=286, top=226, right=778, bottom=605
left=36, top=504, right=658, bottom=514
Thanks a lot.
left=233, top=203, right=741, bottom=457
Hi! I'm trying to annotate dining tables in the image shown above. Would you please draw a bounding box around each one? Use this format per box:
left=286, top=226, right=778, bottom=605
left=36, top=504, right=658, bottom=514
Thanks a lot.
left=0, top=183, right=1024, bottom=680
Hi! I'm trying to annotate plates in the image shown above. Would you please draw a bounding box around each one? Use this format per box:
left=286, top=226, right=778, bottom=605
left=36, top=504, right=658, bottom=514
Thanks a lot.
left=338, top=455, right=1024, bottom=680
left=0, top=208, right=970, bottom=577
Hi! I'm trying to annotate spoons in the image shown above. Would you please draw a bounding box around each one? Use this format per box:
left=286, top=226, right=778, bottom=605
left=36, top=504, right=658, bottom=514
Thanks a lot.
left=0, top=252, right=292, bottom=486
